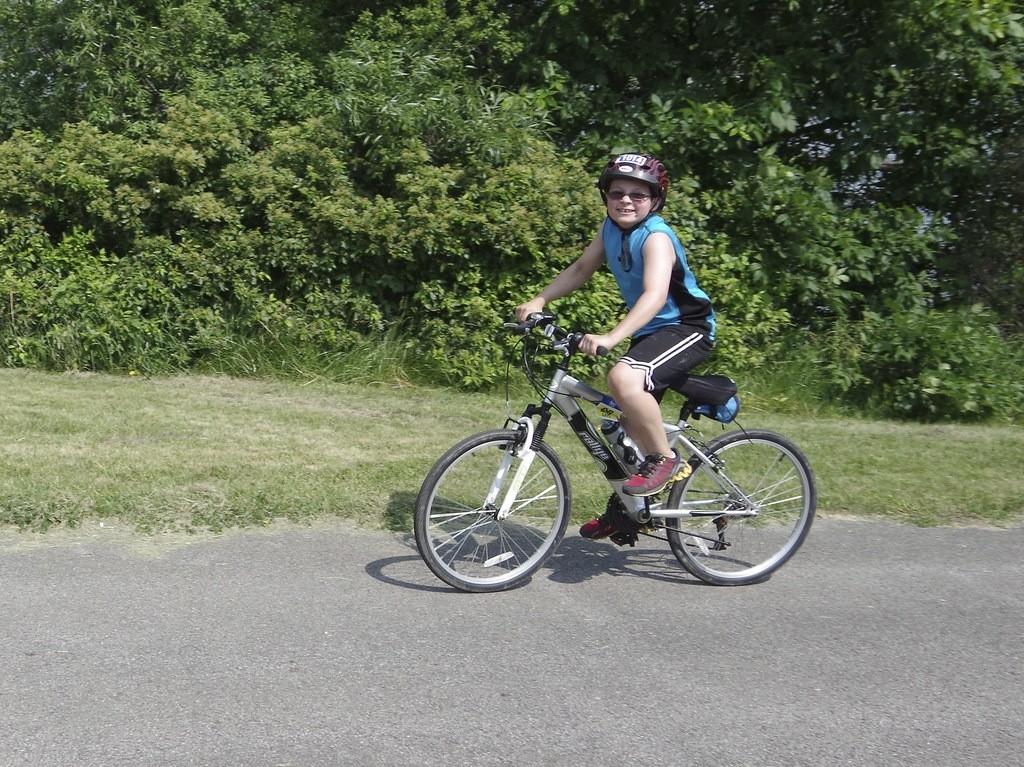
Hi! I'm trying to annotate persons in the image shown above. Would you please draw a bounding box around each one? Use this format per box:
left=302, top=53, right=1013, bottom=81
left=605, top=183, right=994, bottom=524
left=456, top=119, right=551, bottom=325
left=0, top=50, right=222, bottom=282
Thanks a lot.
left=516, top=152, right=717, bottom=541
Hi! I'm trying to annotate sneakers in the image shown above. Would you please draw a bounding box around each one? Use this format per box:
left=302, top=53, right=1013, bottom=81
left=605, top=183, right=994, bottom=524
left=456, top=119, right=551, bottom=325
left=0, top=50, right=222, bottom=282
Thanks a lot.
left=622, top=448, right=693, bottom=497
left=579, top=514, right=618, bottom=541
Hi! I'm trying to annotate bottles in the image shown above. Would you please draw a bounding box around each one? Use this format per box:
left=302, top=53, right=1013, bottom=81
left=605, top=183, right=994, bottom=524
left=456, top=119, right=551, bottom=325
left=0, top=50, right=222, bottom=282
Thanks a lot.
left=601, top=421, right=644, bottom=474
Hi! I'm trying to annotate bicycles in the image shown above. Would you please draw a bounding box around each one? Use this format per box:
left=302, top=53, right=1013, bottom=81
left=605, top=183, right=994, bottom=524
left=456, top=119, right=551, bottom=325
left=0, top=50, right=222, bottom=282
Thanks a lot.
left=412, top=308, right=817, bottom=587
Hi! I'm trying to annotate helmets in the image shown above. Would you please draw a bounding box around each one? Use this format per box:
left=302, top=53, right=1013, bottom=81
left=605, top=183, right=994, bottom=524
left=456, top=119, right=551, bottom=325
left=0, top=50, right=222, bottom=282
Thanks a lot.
left=598, top=152, right=668, bottom=212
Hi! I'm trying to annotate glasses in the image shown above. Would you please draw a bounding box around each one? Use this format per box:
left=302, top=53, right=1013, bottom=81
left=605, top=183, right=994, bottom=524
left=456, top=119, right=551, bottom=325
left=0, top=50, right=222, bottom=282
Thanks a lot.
left=604, top=189, right=653, bottom=202
